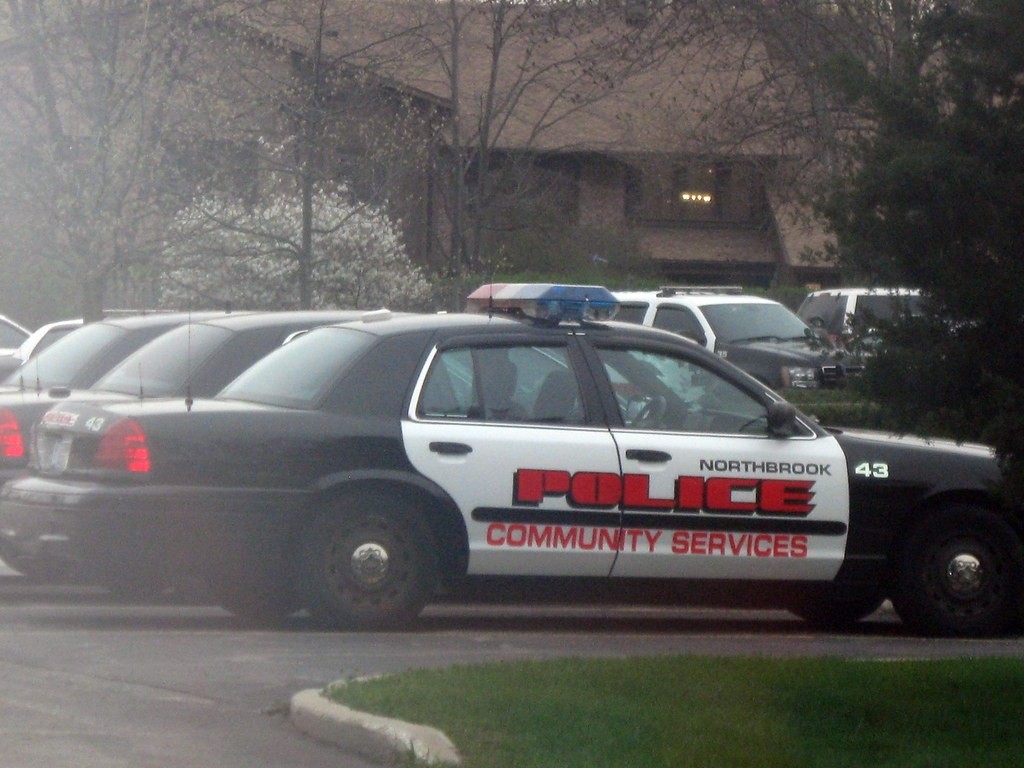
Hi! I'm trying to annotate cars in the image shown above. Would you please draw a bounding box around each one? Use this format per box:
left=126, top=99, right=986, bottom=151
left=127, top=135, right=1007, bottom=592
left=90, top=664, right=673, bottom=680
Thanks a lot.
left=0, top=276, right=1024, bottom=639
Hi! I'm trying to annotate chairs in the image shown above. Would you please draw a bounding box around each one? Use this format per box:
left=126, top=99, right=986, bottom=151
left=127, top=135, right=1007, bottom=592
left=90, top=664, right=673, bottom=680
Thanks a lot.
left=532, top=371, right=577, bottom=422
left=467, top=360, right=527, bottom=420
